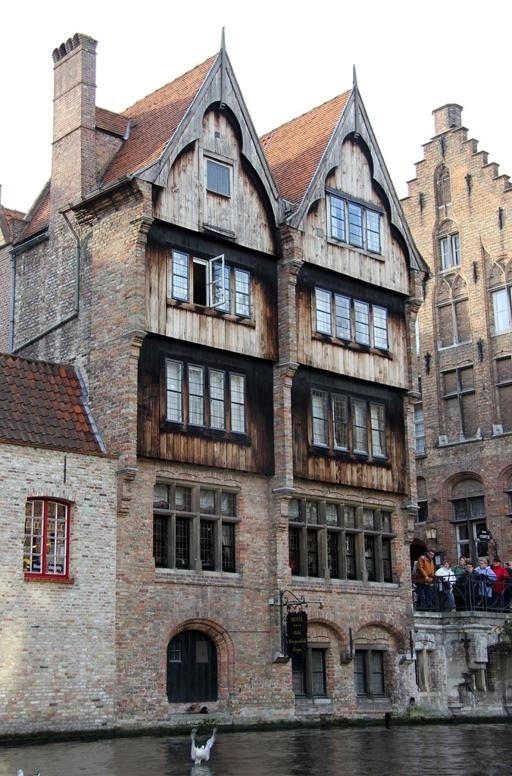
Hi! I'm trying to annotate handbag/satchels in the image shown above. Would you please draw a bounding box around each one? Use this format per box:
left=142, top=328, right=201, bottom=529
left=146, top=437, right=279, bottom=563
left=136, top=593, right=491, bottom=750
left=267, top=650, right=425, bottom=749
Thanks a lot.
left=444, top=581, right=451, bottom=591
left=410, top=561, right=420, bottom=578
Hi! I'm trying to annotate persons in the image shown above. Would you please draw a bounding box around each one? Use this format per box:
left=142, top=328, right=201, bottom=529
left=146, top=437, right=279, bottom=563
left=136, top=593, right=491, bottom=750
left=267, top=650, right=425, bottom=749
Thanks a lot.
left=411, top=549, right=512, bottom=613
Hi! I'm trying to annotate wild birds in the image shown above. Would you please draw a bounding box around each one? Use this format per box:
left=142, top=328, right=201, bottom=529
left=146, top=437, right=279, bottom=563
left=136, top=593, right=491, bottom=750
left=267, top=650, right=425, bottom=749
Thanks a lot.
left=189, top=727, right=217, bottom=767
left=186, top=704, right=198, bottom=713
left=16, top=768, right=41, bottom=776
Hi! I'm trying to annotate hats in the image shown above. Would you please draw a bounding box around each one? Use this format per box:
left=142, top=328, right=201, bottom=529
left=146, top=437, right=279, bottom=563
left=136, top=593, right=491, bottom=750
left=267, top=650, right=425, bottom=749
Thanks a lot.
left=494, top=556, right=501, bottom=562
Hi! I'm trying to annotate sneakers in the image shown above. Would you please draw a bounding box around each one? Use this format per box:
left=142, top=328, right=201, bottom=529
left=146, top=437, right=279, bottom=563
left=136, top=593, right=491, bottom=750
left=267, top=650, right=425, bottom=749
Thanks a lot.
left=451, top=608, right=457, bottom=613
